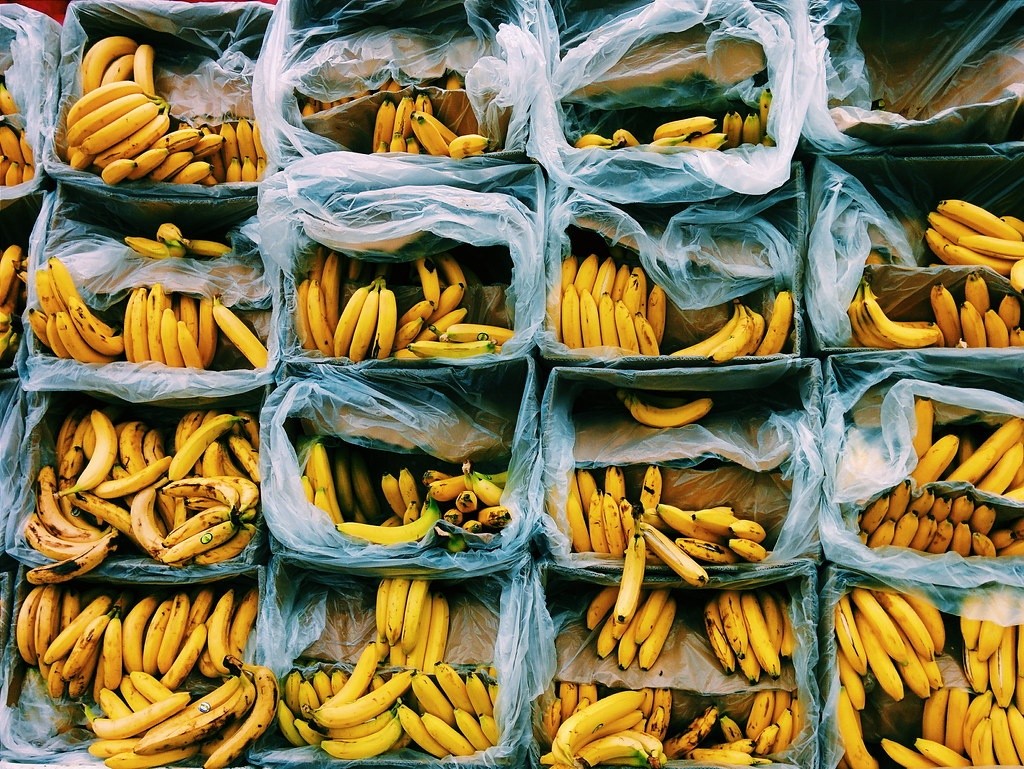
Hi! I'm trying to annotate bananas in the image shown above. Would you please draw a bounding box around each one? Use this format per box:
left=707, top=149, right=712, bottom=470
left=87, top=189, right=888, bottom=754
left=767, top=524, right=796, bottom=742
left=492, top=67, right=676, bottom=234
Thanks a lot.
left=0, top=34, right=1024, bottom=768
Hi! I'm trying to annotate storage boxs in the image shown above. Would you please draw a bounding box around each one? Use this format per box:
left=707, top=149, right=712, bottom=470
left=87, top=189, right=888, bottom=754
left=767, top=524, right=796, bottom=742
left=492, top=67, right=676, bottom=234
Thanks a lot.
left=0, top=0, right=1024, bottom=769
